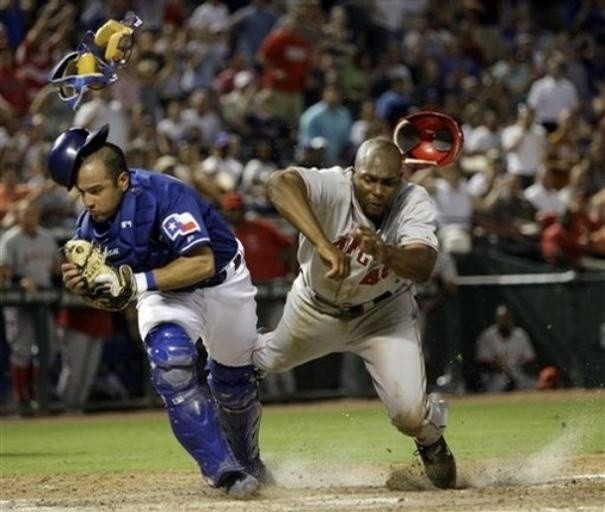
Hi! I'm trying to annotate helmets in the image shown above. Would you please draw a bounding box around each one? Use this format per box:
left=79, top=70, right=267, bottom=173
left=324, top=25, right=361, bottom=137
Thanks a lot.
left=45, top=123, right=110, bottom=191
left=393, top=110, right=464, bottom=169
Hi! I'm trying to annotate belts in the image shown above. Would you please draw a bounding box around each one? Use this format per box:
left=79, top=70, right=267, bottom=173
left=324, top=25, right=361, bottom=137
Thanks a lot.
left=297, top=269, right=390, bottom=319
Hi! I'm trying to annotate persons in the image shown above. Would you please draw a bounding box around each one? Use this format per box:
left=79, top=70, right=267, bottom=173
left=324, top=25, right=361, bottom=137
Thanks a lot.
left=61, top=142, right=277, bottom=501
left=252, top=134, right=457, bottom=491
left=2, top=1, right=605, bottom=418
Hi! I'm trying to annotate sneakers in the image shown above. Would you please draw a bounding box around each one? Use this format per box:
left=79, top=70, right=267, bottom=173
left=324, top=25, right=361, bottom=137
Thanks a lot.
left=221, top=471, right=260, bottom=500
left=245, top=457, right=278, bottom=491
left=413, top=435, right=456, bottom=489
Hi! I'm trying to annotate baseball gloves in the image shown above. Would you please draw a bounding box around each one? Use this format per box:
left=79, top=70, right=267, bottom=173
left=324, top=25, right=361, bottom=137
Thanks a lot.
left=56, top=238, right=136, bottom=312
